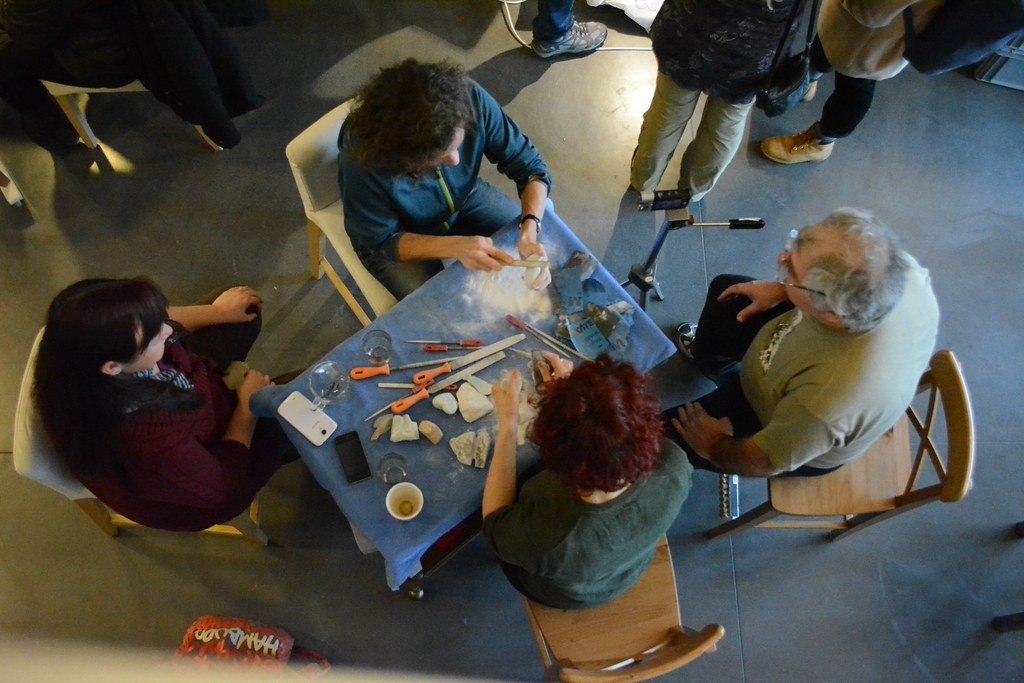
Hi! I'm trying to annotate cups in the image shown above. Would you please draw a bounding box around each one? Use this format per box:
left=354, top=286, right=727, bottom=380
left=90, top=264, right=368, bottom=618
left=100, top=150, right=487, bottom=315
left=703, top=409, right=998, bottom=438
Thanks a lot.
left=362, top=329, right=392, bottom=366
left=376, top=451, right=409, bottom=486
left=385, top=481, right=424, bottom=521
left=309, top=361, right=352, bottom=413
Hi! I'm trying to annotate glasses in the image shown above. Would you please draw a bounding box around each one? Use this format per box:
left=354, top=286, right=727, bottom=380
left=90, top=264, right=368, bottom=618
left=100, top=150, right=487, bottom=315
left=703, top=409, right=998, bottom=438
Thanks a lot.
left=775, top=228, right=828, bottom=297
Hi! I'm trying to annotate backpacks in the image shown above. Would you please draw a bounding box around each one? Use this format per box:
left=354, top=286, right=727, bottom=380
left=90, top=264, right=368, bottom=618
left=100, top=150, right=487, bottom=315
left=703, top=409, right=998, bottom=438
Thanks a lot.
left=903, top=0, right=1024, bottom=75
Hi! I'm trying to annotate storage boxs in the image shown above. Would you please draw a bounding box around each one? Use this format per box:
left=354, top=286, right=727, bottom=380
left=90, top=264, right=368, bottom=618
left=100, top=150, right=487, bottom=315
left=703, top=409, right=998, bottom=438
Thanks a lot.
left=973, top=35, right=1024, bottom=92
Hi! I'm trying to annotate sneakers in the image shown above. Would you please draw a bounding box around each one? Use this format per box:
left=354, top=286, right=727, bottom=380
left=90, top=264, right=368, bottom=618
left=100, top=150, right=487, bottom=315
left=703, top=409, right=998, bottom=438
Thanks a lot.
left=530, top=20, right=607, bottom=58
left=673, top=321, right=699, bottom=362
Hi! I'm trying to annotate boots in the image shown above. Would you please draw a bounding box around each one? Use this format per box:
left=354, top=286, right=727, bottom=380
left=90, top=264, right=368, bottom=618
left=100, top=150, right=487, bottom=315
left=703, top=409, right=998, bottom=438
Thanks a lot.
left=761, top=120, right=836, bottom=165
left=800, top=80, right=818, bottom=103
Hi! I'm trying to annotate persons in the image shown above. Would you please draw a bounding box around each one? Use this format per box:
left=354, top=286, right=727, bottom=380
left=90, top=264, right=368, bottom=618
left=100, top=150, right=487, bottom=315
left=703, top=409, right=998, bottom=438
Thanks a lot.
left=333, top=56, right=554, bottom=302
left=478, top=349, right=696, bottom=611
left=629, top=0, right=822, bottom=204
left=499, top=0, right=608, bottom=59
left=30, top=273, right=349, bottom=533
left=652, top=206, right=942, bottom=481
left=758, top=0, right=949, bottom=166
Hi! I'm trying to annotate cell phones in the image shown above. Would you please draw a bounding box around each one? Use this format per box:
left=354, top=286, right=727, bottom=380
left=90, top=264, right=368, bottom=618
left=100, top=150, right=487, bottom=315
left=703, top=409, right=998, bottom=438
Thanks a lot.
left=334, top=430, right=371, bottom=485
left=277, top=390, right=338, bottom=447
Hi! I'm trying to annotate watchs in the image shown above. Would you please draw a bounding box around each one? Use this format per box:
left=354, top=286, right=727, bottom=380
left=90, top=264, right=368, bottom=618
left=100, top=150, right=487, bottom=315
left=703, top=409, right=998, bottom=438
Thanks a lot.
left=518, top=214, right=541, bottom=234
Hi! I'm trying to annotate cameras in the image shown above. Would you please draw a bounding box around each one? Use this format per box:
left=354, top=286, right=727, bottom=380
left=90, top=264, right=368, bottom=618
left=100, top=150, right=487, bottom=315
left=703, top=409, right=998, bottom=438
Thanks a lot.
left=638, top=189, right=692, bottom=211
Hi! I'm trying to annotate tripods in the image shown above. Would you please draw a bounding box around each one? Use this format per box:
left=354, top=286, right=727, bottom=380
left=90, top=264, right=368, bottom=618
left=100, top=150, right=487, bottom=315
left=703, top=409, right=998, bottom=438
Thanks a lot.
left=619, top=208, right=765, bottom=314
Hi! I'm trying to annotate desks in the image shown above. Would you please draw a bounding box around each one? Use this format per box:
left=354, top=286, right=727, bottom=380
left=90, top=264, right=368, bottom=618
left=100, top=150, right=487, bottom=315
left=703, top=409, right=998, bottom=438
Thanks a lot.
left=247, top=197, right=678, bottom=599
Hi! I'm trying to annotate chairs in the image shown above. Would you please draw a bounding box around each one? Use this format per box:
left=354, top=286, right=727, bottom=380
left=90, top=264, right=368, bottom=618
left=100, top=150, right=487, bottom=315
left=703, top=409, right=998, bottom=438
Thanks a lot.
left=522, top=534, right=726, bottom=683
left=286, top=93, right=458, bottom=329
left=704, top=349, right=978, bottom=542
left=13, top=324, right=268, bottom=547
left=42, top=77, right=226, bottom=152
